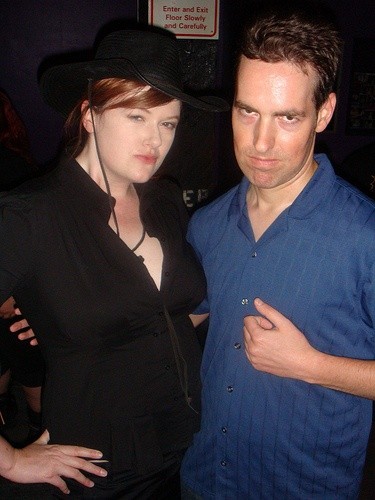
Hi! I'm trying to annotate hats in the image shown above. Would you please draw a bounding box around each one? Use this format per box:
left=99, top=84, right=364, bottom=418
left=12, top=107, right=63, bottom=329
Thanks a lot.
left=39, top=25, right=231, bottom=115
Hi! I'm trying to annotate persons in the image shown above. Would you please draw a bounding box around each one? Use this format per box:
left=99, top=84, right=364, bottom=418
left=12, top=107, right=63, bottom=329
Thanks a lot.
left=0, top=18, right=210, bottom=500
left=0, top=92, right=45, bottom=435
left=10, top=11, right=375, bottom=500
